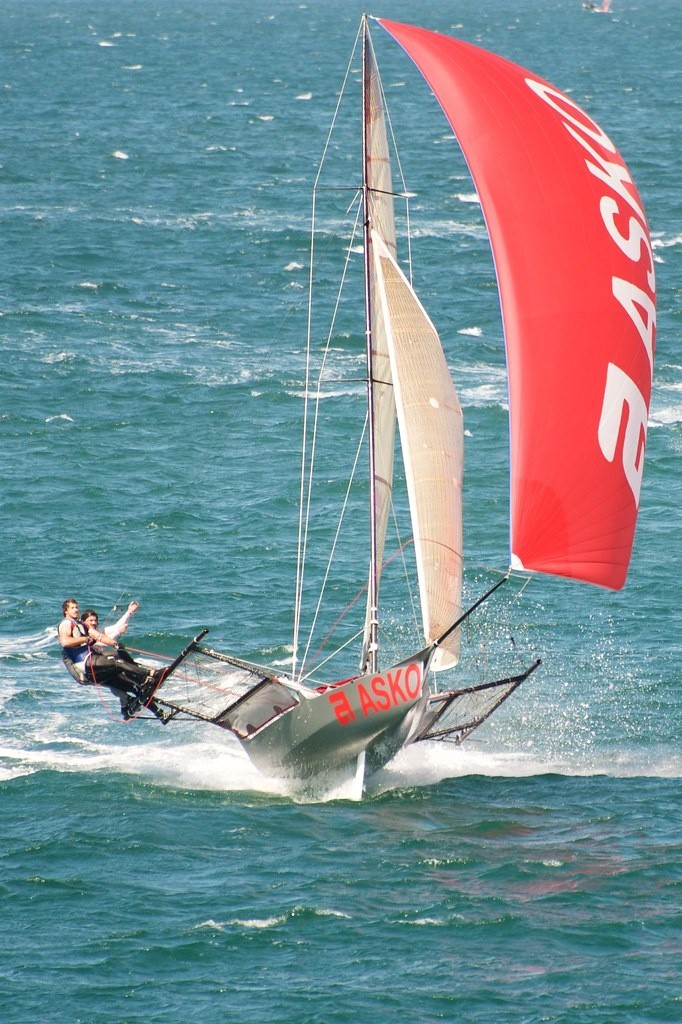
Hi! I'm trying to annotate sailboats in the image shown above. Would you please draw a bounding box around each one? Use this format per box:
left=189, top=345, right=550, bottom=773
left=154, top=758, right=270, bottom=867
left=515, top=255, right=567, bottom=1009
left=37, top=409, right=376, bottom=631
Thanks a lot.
left=96, top=12, right=657, bottom=796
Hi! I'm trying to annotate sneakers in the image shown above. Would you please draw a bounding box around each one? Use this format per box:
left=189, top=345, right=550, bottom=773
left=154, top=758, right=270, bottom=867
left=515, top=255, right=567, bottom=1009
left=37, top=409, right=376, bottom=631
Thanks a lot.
left=121, top=668, right=168, bottom=715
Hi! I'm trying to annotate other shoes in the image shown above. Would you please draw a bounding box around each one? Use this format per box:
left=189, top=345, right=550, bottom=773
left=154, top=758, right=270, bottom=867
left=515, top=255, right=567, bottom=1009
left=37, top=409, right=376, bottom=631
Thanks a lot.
left=159, top=709, right=179, bottom=725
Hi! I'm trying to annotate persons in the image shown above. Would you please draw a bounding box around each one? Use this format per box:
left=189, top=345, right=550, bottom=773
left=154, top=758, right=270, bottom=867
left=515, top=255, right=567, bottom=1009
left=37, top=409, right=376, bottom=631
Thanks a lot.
left=57, top=598, right=176, bottom=724
